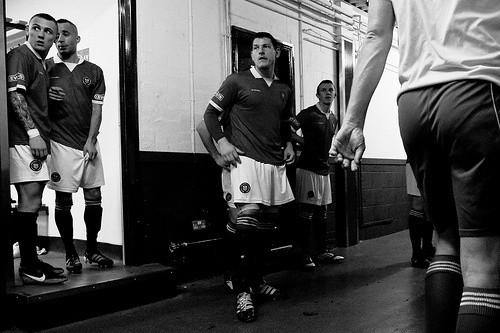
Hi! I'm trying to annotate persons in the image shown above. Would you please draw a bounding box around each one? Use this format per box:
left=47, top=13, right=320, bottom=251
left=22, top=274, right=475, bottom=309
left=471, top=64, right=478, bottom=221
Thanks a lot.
left=327, top=0, right=500, bottom=333
left=204, top=32, right=296, bottom=323
left=5, top=13, right=68, bottom=284
left=9, top=18, right=114, bottom=272
left=405, top=158, right=436, bottom=268
left=196, top=109, right=263, bottom=295
left=292, top=81, right=345, bottom=269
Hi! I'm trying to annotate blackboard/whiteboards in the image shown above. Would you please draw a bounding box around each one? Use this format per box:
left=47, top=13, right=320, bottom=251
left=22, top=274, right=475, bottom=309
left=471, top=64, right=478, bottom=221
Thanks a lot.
left=231, top=25, right=297, bottom=116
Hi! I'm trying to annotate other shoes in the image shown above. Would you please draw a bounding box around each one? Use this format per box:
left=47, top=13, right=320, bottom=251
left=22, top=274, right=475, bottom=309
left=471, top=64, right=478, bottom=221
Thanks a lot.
left=322, top=250, right=344, bottom=262
left=305, top=256, right=316, bottom=266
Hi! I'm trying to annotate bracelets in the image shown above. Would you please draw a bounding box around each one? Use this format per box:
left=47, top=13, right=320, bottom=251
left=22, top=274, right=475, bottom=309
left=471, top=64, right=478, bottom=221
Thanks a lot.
left=296, top=136, right=300, bottom=142
left=27, top=129, right=40, bottom=140
left=88, top=137, right=97, bottom=144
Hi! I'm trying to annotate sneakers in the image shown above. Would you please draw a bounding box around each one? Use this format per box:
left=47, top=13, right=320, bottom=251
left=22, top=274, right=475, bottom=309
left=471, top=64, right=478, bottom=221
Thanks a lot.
left=66, top=254, right=82, bottom=270
left=422, top=245, right=437, bottom=260
left=412, top=255, right=430, bottom=269
left=84, top=249, right=113, bottom=267
left=255, top=280, right=284, bottom=299
left=19, top=270, right=68, bottom=285
left=39, top=260, right=64, bottom=273
left=236, top=289, right=256, bottom=322
